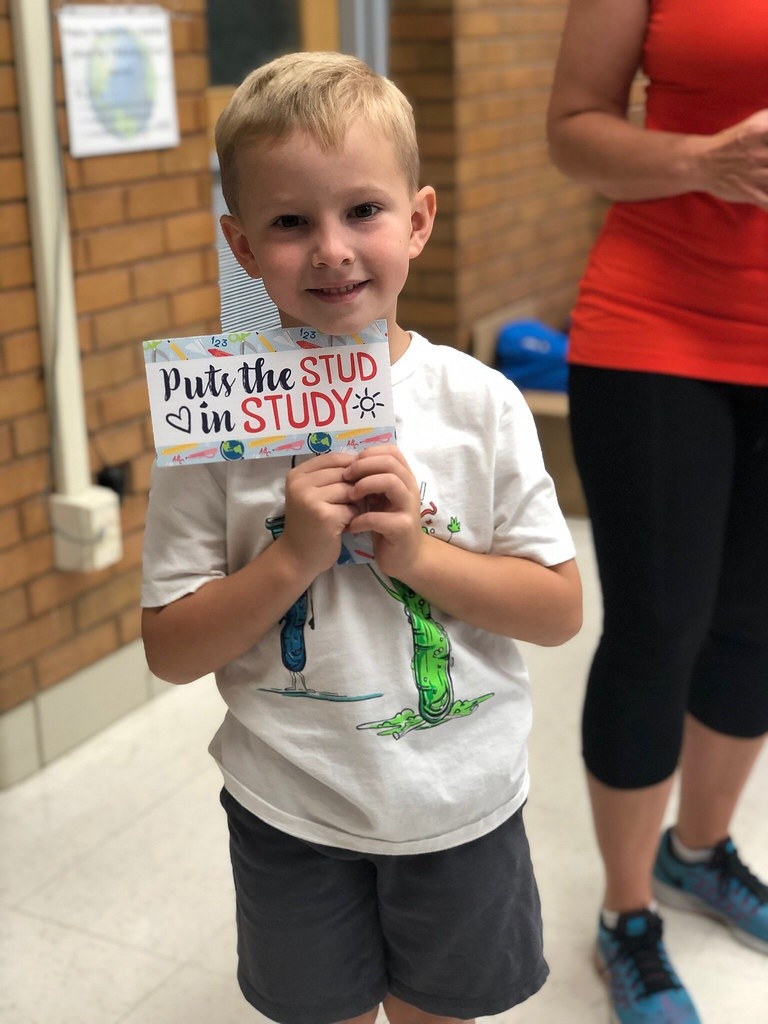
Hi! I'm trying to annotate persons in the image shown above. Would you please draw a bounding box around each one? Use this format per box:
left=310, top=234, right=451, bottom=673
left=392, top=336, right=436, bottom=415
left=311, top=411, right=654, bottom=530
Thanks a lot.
left=550, top=0, right=768, bottom=1024
left=140, top=50, right=585, bottom=1024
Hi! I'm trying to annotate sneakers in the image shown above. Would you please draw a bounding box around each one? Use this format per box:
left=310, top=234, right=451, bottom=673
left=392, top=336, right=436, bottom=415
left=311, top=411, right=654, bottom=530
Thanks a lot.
left=650, top=825, right=768, bottom=955
left=591, top=907, right=700, bottom=1024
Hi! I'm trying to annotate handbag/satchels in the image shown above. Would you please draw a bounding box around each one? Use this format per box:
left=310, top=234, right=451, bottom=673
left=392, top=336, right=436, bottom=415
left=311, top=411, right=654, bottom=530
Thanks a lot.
left=496, top=319, right=572, bottom=393
